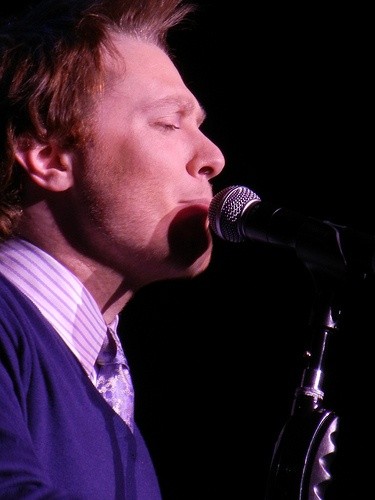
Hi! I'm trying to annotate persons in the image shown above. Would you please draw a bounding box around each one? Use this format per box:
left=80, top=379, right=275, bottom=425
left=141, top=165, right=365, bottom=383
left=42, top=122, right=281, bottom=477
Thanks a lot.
left=1, top=1, right=226, bottom=500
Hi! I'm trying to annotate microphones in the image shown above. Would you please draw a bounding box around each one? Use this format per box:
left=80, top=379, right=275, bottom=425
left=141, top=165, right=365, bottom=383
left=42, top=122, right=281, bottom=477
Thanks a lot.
left=209, top=183, right=375, bottom=285
